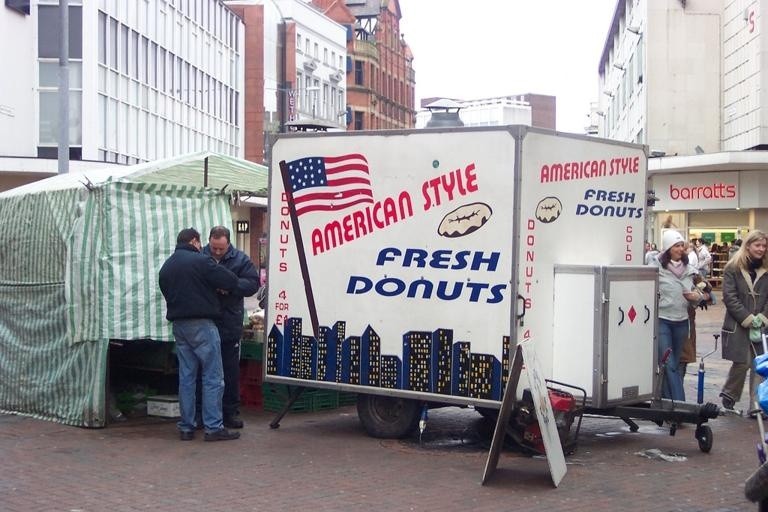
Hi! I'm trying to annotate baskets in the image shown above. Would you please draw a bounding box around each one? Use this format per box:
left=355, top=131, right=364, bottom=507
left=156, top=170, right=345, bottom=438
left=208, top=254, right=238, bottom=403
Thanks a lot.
left=239, top=340, right=358, bottom=414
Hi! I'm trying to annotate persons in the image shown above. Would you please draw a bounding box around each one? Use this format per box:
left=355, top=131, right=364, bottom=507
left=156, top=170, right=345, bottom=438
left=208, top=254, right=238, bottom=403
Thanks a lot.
left=159, top=227, right=241, bottom=440
left=195, top=225, right=259, bottom=430
left=644, top=228, right=768, bottom=410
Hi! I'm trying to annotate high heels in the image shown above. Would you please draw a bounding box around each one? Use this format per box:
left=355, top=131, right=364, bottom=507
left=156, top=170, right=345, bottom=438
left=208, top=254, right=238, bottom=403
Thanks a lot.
left=719, top=392, right=735, bottom=410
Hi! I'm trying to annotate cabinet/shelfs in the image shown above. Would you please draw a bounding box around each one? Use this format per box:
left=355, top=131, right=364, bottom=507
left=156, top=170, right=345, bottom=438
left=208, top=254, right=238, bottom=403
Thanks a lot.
left=705, top=252, right=728, bottom=290
left=552, top=264, right=658, bottom=410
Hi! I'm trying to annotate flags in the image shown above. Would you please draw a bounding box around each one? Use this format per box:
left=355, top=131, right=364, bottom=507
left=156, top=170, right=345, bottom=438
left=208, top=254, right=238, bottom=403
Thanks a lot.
left=286, top=154, right=374, bottom=216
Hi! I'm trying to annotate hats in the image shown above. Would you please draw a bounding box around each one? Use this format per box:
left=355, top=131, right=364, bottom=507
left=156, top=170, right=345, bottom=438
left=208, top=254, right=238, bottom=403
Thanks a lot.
left=661, top=229, right=685, bottom=256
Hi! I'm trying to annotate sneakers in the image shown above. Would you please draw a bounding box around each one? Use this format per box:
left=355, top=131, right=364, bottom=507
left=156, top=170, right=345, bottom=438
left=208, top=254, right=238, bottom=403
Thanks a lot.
left=180, top=431, right=194, bottom=441
left=194, top=414, right=204, bottom=429
left=205, top=428, right=240, bottom=442
left=224, top=413, right=242, bottom=428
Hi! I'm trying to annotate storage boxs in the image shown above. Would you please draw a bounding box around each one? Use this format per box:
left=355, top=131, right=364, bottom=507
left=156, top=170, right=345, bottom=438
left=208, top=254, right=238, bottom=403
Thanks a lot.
left=147, top=394, right=181, bottom=417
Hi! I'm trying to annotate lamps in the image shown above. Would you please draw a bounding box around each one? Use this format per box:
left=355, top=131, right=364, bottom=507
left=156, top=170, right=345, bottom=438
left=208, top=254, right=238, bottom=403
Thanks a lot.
left=627, top=26, right=642, bottom=35
left=338, top=111, right=348, bottom=117
left=614, top=64, right=626, bottom=70
left=604, top=90, right=615, bottom=97
left=596, top=111, right=605, bottom=117
left=264, top=86, right=320, bottom=91
